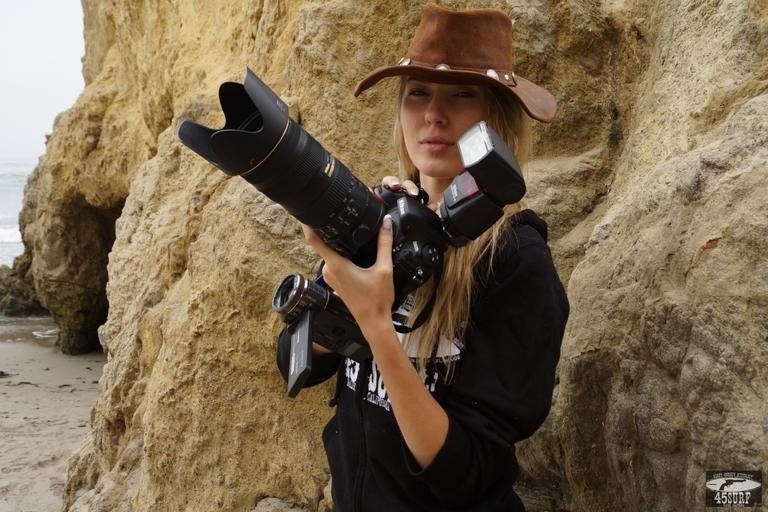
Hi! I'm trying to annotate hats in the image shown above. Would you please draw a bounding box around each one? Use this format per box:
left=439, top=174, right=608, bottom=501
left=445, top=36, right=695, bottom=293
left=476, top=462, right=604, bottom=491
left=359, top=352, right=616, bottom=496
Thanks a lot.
left=355, top=1, right=557, bottom=123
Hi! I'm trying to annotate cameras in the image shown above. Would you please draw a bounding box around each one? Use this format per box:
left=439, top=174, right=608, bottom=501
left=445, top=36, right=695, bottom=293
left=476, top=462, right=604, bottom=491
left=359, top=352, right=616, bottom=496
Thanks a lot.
left=178, top=68, right=528, bottom=311
left=269, top=271, right=376, bottom=398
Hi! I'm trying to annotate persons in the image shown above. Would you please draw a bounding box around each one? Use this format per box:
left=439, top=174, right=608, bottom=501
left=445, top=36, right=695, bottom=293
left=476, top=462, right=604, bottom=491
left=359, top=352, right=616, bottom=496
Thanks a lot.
left=274, top=0, right=572, bottom=512
